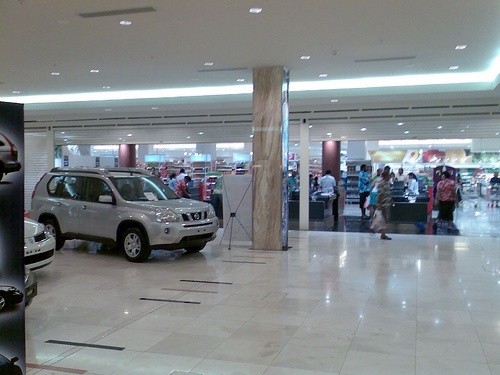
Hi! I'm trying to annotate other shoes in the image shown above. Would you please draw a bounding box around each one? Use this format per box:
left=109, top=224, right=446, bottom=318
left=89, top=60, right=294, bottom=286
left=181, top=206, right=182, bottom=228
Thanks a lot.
left=381, top=235, right=391, bottom=240
left=362, top=215, right=369, bottom=219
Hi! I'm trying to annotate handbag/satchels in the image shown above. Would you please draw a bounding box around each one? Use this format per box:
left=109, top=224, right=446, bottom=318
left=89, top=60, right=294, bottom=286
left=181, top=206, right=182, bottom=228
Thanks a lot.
left=369, top=208, right=388, bottom=233
left=363, top=195, right=371, bottom=208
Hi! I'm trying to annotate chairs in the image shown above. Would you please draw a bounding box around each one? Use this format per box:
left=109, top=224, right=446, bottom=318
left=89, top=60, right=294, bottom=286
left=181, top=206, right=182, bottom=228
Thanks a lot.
left=93, top=182, right=107, bottom=199
left=121, top=184, right=136, bottom=201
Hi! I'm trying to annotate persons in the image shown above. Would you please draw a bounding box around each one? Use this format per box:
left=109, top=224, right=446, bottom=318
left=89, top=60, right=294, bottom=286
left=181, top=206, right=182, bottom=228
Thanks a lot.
left=435, top=171, right=458, bottom=222
left=167, top=168, right=192, bottom=199
left=489, top=170, right=500, bottom=208
left=395, top=167, right=418, bottom=193
left=359, top=161, right=396, bottom=240
left=317, top=169, right=337, bottom=194
left=287, top=171, right=297, bottom=188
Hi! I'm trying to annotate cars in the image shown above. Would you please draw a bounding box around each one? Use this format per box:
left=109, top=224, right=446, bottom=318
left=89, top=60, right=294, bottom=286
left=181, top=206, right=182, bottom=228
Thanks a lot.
left=0, top=132, right=21, bottom=181
left=0, top=354, right=22, bottom=375
left=24, top=217, right=56, bottom=309
left=0, top=285, right=23, bottom=312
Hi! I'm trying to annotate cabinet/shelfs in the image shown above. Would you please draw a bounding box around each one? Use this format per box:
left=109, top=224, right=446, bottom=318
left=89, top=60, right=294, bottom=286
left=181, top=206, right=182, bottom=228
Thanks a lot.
left=165, top=164, right=206, bottom=179
left=343, top=160, right=378, bottom=204
left=212, top=162, right=248, bottom=175
left=288, top=160, right=321, bottom=178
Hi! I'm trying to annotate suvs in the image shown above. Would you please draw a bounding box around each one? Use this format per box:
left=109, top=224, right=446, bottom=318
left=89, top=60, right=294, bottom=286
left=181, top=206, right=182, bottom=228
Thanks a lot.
left=29, top=165, right=219, bottom=263
